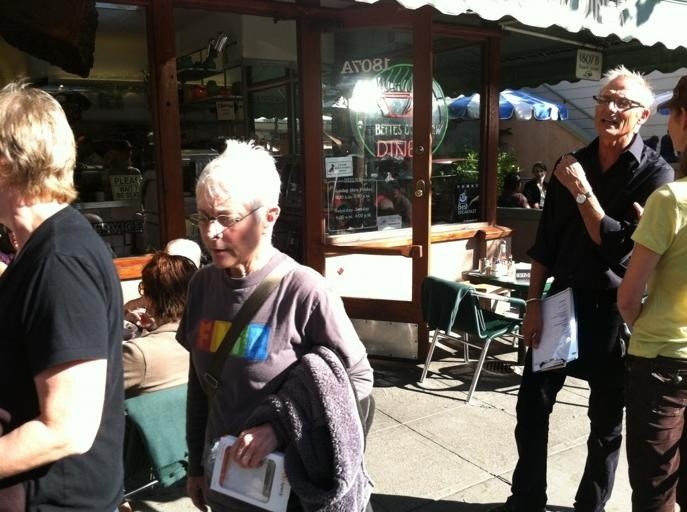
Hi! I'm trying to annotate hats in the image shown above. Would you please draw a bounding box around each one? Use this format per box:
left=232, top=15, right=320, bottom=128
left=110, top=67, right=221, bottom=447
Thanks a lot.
left=164, top=238, right=202, bottom=269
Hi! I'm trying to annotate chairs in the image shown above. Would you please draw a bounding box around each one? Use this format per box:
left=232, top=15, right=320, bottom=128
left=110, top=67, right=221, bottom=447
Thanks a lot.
left=420, top=275, right=527, bottom=403
left=122, top=382, right=188, bottom=512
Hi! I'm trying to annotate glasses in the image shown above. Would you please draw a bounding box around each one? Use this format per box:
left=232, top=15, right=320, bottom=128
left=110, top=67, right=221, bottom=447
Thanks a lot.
left=138, top=282, right=146, bottom=296
left=590, top=93, right=644, bottom=109
left=186, top=204, right=266, bottom=229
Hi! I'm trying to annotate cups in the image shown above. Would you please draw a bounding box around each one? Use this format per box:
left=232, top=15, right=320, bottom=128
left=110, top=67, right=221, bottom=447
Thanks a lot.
left=479, top=258, right=486, bottom=274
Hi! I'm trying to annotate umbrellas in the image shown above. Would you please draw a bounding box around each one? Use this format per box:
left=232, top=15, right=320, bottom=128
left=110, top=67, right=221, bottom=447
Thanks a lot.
left=446, top=87, right=569, bottom=126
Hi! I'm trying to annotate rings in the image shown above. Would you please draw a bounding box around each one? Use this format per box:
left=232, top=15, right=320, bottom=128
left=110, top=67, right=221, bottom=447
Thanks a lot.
left=562, top=155, right=571, bottom=162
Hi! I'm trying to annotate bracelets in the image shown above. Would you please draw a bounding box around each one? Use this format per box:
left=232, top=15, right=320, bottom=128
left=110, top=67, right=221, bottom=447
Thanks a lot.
left=521, top=296, right=542, bottom=306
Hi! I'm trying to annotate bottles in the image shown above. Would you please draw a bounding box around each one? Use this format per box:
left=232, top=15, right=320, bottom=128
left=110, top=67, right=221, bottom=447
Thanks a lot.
left=497, top=240, right=507, bottom=263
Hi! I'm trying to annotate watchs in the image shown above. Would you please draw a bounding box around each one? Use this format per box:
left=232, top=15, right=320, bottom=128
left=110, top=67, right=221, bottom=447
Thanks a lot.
left=567, top=189, right=599, bottom=207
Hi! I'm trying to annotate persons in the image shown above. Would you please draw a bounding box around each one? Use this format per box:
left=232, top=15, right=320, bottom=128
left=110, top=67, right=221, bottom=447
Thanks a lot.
left=611, top=74, right=687, bottom=512
left=0, top=72, right=130, bottom=512
left=122, top=252, right=198, bottom=396
left=173, top=135, right=376, bottom=512
left=499, top=167, right=534, bottom=209
left=123, top=237, right=204, bottom=329
left=524, top=159, right=550, bottom=210
left=498, top=62, right=677, bottom=512
